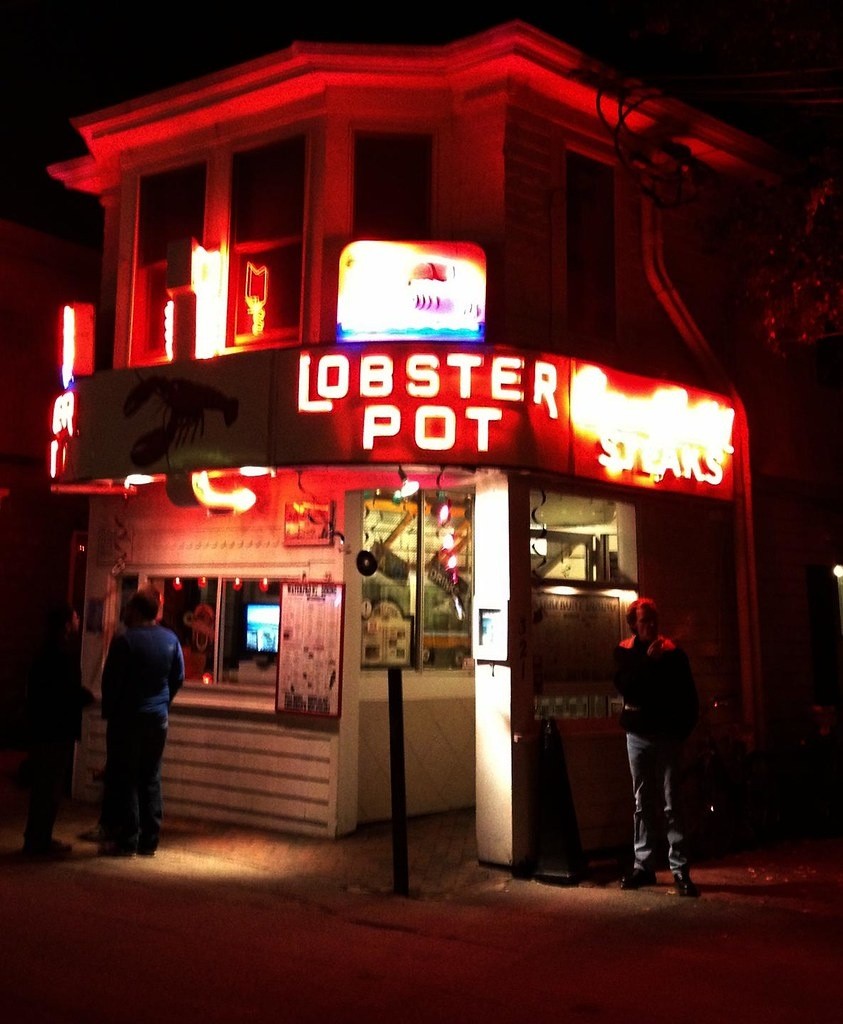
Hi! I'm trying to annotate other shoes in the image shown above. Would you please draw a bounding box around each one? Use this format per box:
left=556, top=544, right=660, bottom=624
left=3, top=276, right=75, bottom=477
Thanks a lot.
left=22, top=837, right=71, bottom=862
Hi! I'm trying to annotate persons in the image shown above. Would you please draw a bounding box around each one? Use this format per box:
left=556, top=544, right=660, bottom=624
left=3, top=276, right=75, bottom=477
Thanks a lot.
left=98, top=588, right=186, bottom=856
left=606, top=595, right=704, bottom=897
left=19, top=603, right=104, bottom=857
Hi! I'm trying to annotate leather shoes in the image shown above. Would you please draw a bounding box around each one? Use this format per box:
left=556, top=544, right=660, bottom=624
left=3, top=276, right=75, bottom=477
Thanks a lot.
left=620, top=874, right=657, bottom=889
left=674, top=872, right=700, bottom=897
